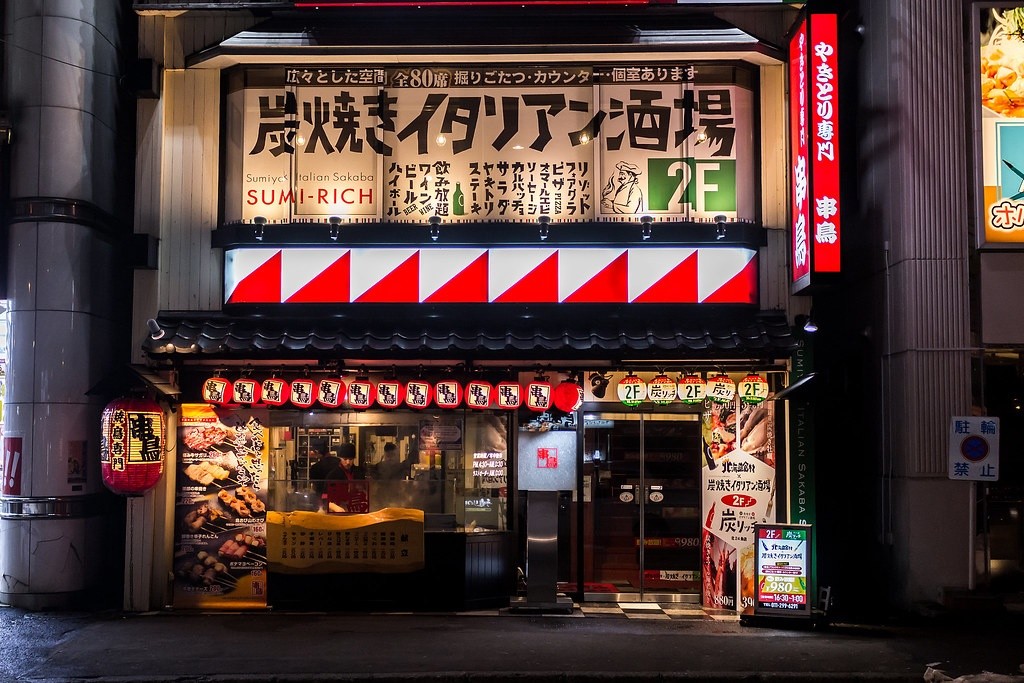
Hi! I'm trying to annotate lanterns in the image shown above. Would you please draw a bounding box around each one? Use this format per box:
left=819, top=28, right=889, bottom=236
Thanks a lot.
left=202, top=376, right=586, bottom=414
left=102, top=397, right=167, bottom=499
left=617, top=374, right=768, bottom=406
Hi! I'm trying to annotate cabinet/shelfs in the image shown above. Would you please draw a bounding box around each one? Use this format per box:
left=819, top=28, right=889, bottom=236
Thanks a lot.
left=292, top=425, right=364, bottom=489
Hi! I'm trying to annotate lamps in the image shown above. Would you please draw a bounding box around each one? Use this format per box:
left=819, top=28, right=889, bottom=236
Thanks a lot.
left=251, top=216, right=728, bottom=239
left=803, top=317, right=818, bottom=332
left=592, top=370, right=614, bottom=398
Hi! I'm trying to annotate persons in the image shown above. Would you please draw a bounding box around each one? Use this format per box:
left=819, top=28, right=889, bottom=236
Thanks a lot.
left=369, top=435, right=418, bottom=513
left=309, top=439, right=367, bottom=514
